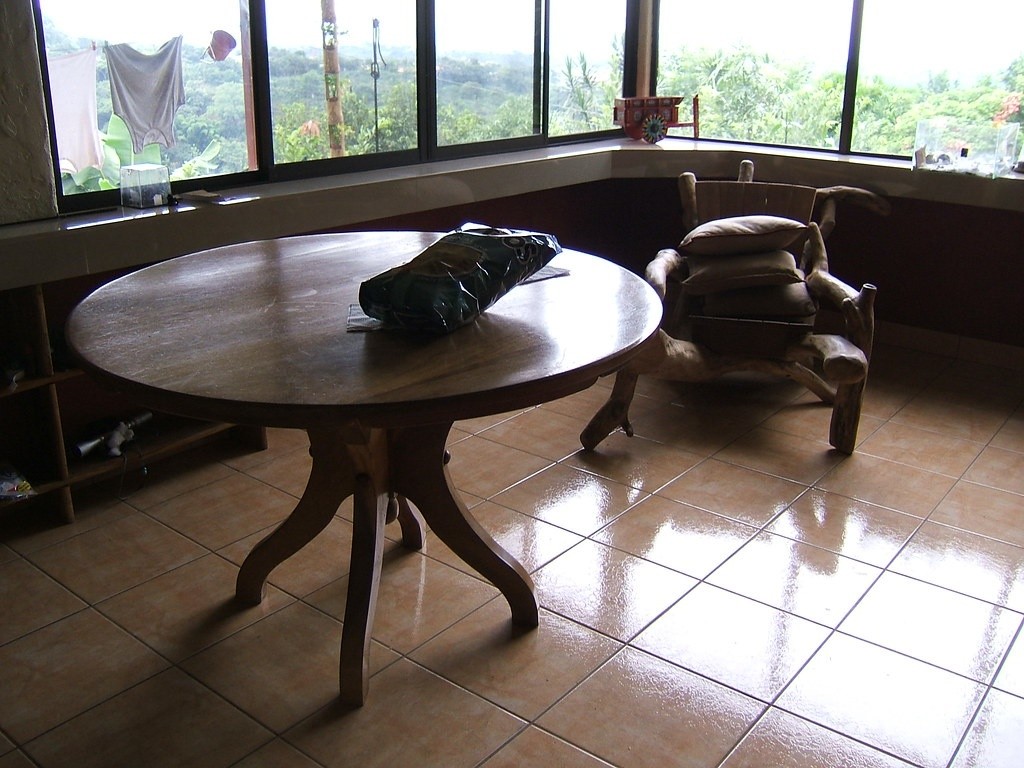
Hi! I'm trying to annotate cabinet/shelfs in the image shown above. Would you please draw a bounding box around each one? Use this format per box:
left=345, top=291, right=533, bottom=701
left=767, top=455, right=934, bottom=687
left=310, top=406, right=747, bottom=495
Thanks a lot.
left=0, top=240, right=272, bottom=527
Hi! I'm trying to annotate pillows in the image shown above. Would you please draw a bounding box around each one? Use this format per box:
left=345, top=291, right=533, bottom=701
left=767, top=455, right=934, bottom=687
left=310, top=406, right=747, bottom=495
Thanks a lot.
left=678, top=216, right=809, bottom=256
left=690, top=281, right=816, bottom=318
left=684, top=251, right=805, bottom=291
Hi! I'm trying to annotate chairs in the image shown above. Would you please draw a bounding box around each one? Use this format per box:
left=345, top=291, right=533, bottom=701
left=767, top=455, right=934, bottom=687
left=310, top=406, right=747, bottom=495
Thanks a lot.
left=578, top=172, right=877, bottom=456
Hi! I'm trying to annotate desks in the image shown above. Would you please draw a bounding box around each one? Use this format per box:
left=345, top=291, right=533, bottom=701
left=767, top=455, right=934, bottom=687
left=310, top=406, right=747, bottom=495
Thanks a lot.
left=69, top=230, right=666, bottom=708
